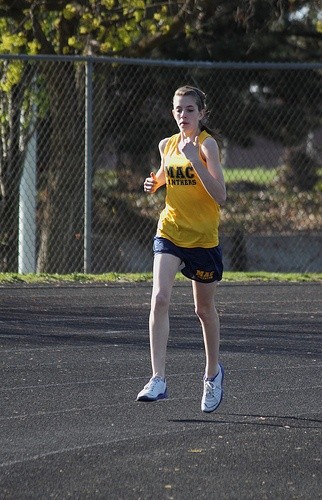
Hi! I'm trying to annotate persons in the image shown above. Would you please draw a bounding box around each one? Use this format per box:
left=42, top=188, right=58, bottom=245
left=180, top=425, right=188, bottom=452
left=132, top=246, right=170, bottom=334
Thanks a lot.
left=136, top=85, right=227, bottom=414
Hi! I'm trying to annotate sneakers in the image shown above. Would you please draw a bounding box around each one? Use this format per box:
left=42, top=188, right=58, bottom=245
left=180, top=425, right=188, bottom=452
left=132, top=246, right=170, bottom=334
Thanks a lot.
left=136, top=372, right=168, bottom=401
left=201, top=364, right=224, bottom=413
